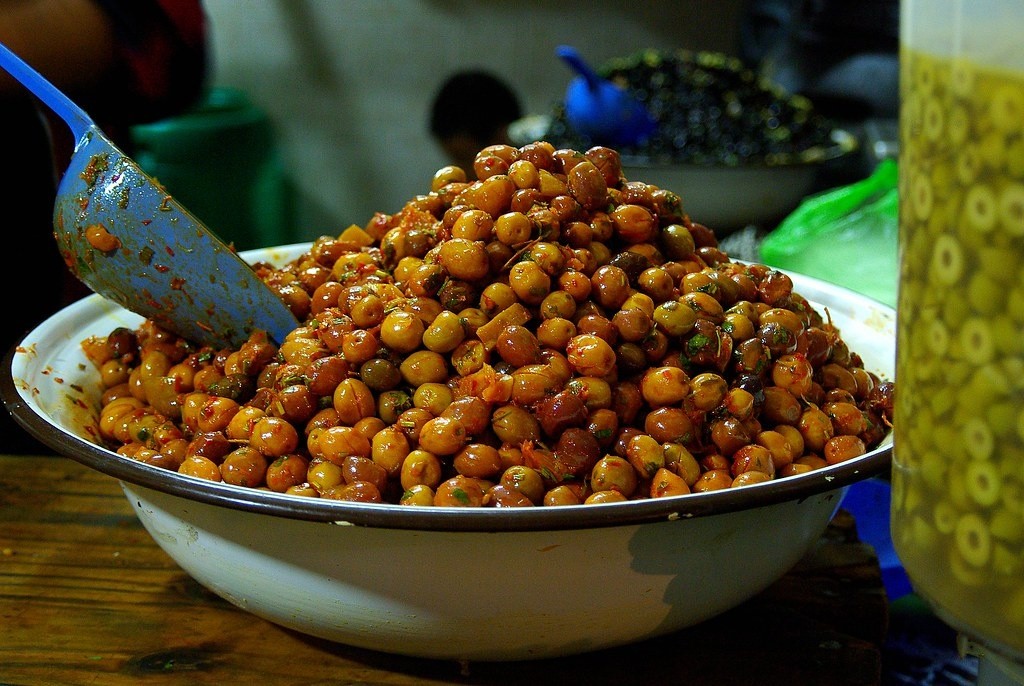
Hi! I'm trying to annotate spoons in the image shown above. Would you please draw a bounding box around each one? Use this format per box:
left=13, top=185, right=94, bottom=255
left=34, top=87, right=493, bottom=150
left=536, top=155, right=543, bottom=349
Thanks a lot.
left=0, top=48, right=299, bottom=347
left=554, top=50, right=659, bottom=145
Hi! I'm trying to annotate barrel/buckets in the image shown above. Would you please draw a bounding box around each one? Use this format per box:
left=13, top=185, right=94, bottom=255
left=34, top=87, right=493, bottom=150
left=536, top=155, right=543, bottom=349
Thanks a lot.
left=127, top=84, right=292, bottom=253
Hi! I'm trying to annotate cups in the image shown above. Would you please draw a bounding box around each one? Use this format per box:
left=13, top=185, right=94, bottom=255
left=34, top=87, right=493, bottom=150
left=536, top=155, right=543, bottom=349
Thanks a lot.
left=888, top=0, right=1024, bottom=654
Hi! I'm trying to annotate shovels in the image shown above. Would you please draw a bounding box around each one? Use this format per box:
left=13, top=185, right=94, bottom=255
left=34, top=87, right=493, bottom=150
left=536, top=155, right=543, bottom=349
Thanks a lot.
left=555, top=43, right=657, bottom=150
left=1, top=43, right=305, bottom=353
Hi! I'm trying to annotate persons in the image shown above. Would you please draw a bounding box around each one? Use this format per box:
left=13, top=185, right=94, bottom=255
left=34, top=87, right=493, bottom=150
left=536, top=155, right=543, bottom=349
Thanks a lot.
left=0, top=0, right=213, bottom=346
left=430, top=68, right=524, bottom=174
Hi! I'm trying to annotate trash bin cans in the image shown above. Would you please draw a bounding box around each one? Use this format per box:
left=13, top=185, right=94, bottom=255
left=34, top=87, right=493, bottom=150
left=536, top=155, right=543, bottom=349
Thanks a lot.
left=124, top=84, right=288, bottom=252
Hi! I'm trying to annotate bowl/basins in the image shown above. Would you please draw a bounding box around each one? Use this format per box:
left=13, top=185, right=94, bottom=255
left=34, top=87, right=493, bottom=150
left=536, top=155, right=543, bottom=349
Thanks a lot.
left=8, top=236, right=904, bottom=663
left=506, top=109, right=864, bottom=237
